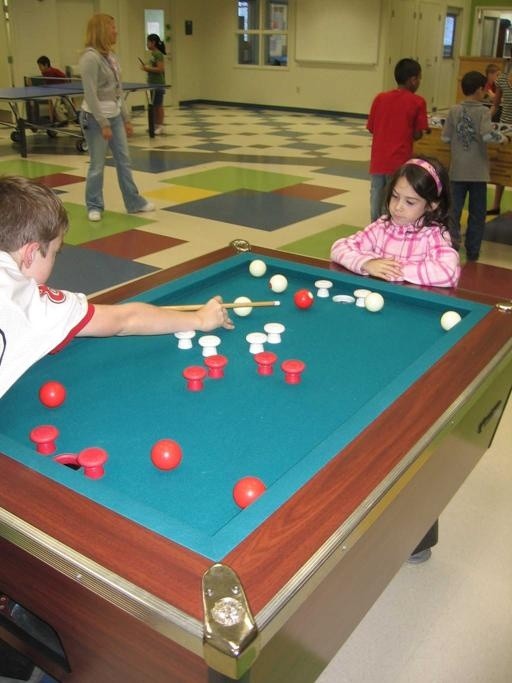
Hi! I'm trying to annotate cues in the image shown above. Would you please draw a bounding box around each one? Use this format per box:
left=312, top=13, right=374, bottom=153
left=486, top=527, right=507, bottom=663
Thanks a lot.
left=158, top=301, right=282, bottom=313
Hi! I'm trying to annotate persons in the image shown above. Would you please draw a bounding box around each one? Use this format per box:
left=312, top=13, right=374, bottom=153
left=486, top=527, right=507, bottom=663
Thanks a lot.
left=0, top=176, right=236, bottom=399
left=142, top=34, right=167, bottom=135
left=37, top=56, right=68, bottom=122
left=329, top=157, right=461, bottom=288
left=487, top=62, right=512, bottom=214
left=440, top=71, right=508, bottom=261
left=80, top=14, right=156, bottom=221
left=485, top=64, right=503, bottom=102
left=367, top=59, right=428, bottom=224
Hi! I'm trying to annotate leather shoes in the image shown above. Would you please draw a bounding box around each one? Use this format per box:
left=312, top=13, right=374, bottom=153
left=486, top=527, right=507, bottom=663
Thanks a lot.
left=487, top=209, right=500, bottom=215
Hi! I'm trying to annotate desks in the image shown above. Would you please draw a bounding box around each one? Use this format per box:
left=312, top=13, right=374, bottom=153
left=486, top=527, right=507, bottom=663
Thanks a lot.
left=1, top=235, right=512, bottom=682
left=0, top=80, right=173, bottom=157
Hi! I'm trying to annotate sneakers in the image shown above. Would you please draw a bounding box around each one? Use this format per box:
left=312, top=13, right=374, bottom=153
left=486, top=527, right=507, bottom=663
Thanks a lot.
left=88, top=212, right=101, bottom=221
left=139, top=204, right=154, bottom=211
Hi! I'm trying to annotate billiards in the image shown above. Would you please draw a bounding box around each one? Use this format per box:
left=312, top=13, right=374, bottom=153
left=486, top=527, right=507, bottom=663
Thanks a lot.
left=269, top=274, right=287, bottom=293
left=441, top=311, right=462, bottom=331
left=40, top=382, right=66, bottom=408
left=233, top=476, right=266, bottom=509
left=249, top=260, right=267, bottom=277
left=294, top=290, right=314, bottom=309
left=365, top=293, right=384, bottom=312
left=232, top=296, right=253, bottom=316
left=151, top=439, right=182, bottom=471
